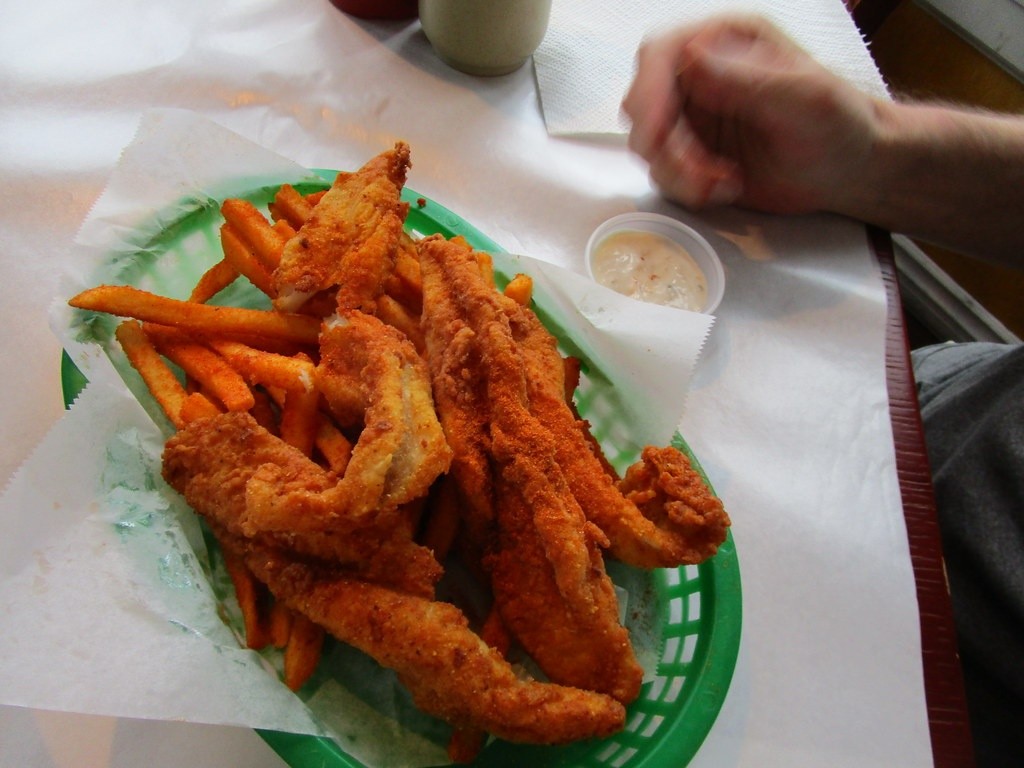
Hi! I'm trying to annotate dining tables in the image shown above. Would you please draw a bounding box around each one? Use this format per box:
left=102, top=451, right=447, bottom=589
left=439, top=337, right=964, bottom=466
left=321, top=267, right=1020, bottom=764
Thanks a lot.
left=0, top=0, right=966, bottom=767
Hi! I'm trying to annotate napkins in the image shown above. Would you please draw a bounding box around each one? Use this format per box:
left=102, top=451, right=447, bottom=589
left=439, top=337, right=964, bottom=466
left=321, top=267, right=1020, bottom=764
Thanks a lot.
left=530, top=0, right=893, bottom=134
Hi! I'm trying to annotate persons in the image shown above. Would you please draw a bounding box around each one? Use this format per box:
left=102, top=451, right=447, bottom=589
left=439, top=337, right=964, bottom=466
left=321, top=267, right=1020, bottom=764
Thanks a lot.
left=621, top=11, right=1024, bottom=768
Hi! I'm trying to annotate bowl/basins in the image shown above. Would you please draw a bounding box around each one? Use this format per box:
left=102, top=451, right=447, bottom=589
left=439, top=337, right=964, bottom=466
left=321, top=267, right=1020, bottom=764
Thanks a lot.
left=583, top=213, right=726, bottom=317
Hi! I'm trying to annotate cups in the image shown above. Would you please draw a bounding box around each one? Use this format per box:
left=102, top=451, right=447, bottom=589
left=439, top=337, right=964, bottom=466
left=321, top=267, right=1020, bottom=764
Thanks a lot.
left=417, top=0, right=553, bottom=78
left=331, top=0, right=420, bottom=19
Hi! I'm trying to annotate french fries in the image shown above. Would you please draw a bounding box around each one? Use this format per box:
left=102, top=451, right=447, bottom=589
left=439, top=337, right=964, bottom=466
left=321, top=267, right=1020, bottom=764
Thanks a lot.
left=65, top=186, right=581, bottom=765
